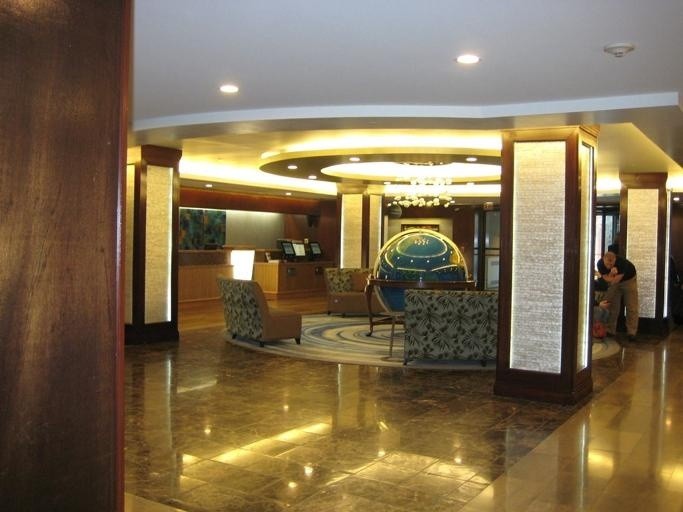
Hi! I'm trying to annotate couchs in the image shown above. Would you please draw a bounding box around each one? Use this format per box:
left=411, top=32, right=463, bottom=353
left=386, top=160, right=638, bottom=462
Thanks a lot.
left=324, top=266, right=381, bottom=318
left=214, top=275, right=300, bottom=348
left=403, top=289, right=499, bottom=366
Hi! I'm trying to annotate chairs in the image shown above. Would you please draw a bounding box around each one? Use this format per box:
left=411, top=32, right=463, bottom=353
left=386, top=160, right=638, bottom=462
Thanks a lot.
left=591, top=288, right=622, bottom=352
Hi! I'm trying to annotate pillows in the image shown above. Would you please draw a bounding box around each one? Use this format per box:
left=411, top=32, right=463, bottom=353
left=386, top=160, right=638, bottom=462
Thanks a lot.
left=349, top=271, right=371, bottom=292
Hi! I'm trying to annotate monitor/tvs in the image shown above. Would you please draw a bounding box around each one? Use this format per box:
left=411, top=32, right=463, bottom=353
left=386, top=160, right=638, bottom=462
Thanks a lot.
left=309, top=241, right=323, bottom=257
left=281, top=241, right=296, bottom=256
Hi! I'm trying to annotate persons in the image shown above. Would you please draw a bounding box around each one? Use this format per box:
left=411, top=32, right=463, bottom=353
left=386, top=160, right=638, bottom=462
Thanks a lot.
left=593, top=233, right=639, bottom=342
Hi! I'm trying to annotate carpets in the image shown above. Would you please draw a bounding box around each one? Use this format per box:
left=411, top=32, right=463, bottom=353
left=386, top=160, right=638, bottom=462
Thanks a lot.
left=219, top=312, right=622, bottom=369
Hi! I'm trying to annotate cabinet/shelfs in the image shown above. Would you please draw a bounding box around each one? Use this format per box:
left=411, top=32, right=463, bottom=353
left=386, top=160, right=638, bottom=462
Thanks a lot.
left=252, top=262, right=304, bottom=296
left=304, top=261, right=336, bottom=294
